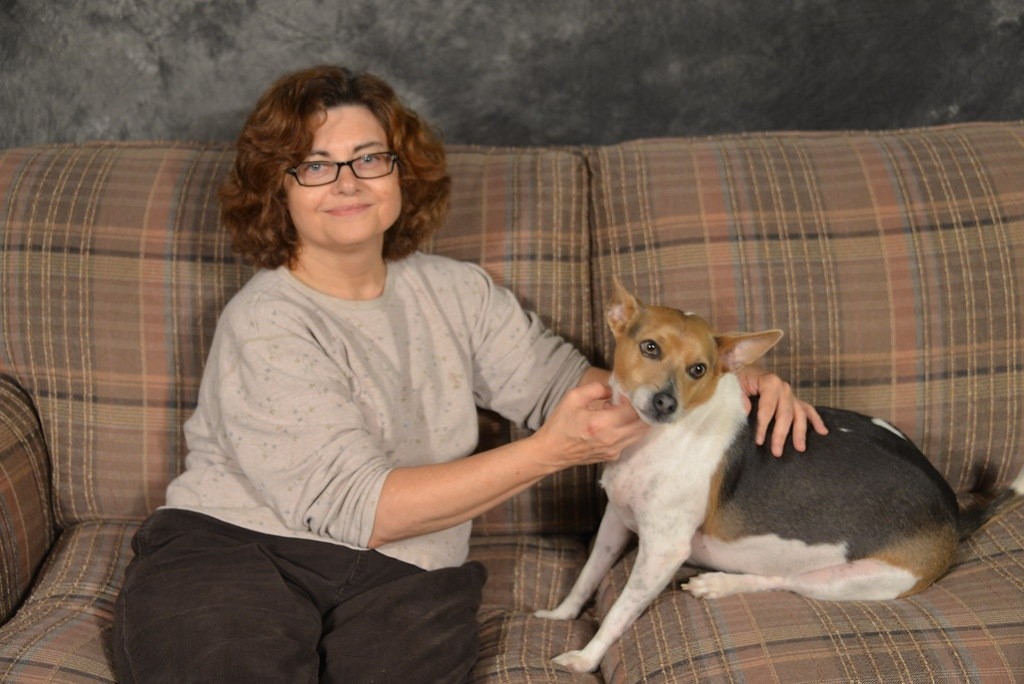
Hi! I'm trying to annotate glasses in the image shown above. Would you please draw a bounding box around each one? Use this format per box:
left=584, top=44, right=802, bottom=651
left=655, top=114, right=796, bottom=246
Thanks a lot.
left=284, top=152, right=399, bottom=187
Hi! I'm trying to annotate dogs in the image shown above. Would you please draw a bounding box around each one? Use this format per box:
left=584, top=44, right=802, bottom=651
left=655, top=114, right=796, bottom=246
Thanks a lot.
left=534, top=273, right=1024, bottom=673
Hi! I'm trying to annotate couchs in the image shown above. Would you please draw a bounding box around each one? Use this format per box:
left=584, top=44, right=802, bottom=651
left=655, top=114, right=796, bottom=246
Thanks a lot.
left=0, top=119, right=1024, bottom=684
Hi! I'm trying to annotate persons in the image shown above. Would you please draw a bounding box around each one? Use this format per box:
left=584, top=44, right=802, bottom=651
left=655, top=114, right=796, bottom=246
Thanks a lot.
left=113, top=65, right=830, bottom=684
